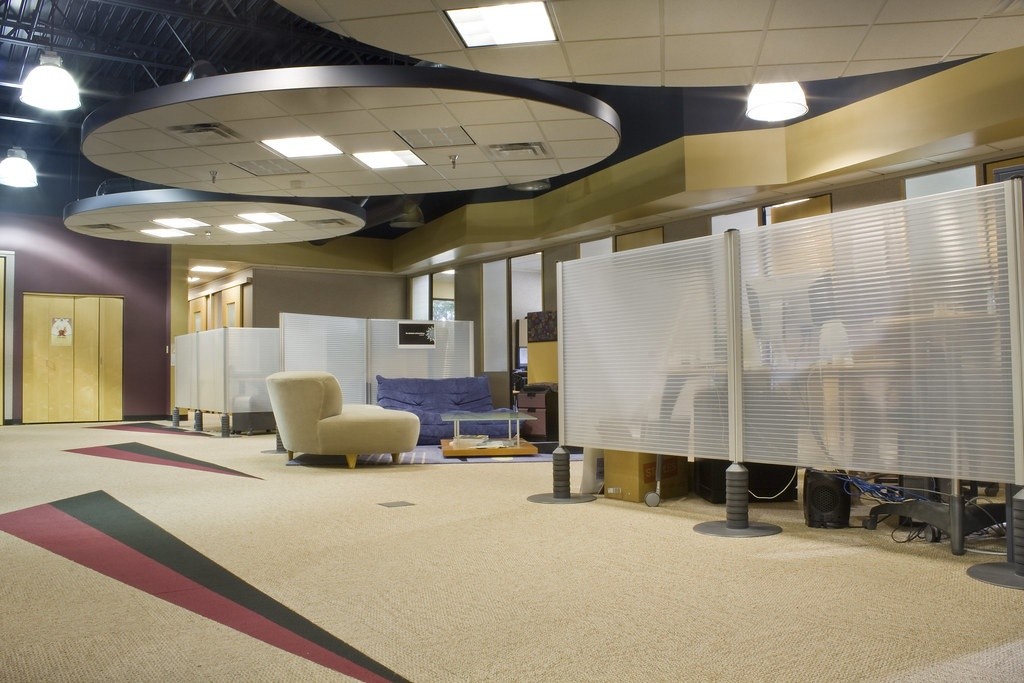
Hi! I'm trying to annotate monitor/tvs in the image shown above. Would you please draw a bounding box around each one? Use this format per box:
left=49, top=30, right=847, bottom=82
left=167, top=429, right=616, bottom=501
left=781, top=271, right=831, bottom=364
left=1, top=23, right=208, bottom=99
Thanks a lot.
left=518, top=346, right=528, bottom=372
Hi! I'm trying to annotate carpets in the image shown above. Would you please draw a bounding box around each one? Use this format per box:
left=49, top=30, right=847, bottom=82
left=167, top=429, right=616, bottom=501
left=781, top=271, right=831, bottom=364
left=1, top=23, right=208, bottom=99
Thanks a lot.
left=285, top=440, right=584, bottom=466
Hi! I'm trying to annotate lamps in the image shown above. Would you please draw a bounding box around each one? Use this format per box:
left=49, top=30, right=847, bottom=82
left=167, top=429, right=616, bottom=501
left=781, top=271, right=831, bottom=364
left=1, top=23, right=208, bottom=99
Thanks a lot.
left=385, top=193, right=426, bottom=229
left=504, top=178, right=551, bottom=191
left=0, top=118, right=39, bottom=190
left=745, top=82, right=810, bottom=124
left=19, top=1, right=82, bottom=113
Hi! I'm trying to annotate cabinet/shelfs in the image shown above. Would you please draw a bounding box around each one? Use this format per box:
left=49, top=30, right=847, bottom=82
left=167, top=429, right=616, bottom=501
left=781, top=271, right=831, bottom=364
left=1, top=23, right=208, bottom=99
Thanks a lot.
left=516, top=383, right=559, bottom=440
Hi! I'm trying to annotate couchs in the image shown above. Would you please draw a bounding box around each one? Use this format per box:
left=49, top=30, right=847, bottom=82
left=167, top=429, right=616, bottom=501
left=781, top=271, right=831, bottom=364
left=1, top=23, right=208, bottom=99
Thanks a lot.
left=375, top=374, right=524, bottom=445
left=265, top=369, right=420, bottom=469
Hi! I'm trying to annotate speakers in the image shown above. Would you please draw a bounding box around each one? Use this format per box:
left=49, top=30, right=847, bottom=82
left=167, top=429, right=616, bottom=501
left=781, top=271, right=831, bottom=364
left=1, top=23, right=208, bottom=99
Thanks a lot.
left=802, top=469, right=850, bottom=529
left=693, top=388, right=797, bottom=502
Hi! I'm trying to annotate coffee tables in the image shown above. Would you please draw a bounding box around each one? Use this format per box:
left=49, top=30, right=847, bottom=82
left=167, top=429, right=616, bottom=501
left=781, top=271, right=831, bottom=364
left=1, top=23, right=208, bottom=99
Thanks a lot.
left=439, top=411, right=538, bottom=458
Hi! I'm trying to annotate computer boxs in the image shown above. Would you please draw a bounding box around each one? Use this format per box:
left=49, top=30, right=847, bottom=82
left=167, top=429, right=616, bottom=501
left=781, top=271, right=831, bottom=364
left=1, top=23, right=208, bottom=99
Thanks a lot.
left=900, top=424, right=936, bottom=527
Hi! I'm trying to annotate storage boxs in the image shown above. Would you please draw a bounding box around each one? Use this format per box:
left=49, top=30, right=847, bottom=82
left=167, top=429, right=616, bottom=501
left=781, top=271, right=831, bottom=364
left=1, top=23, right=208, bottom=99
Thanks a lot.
left=603, top=447, right=689, bottom=503
